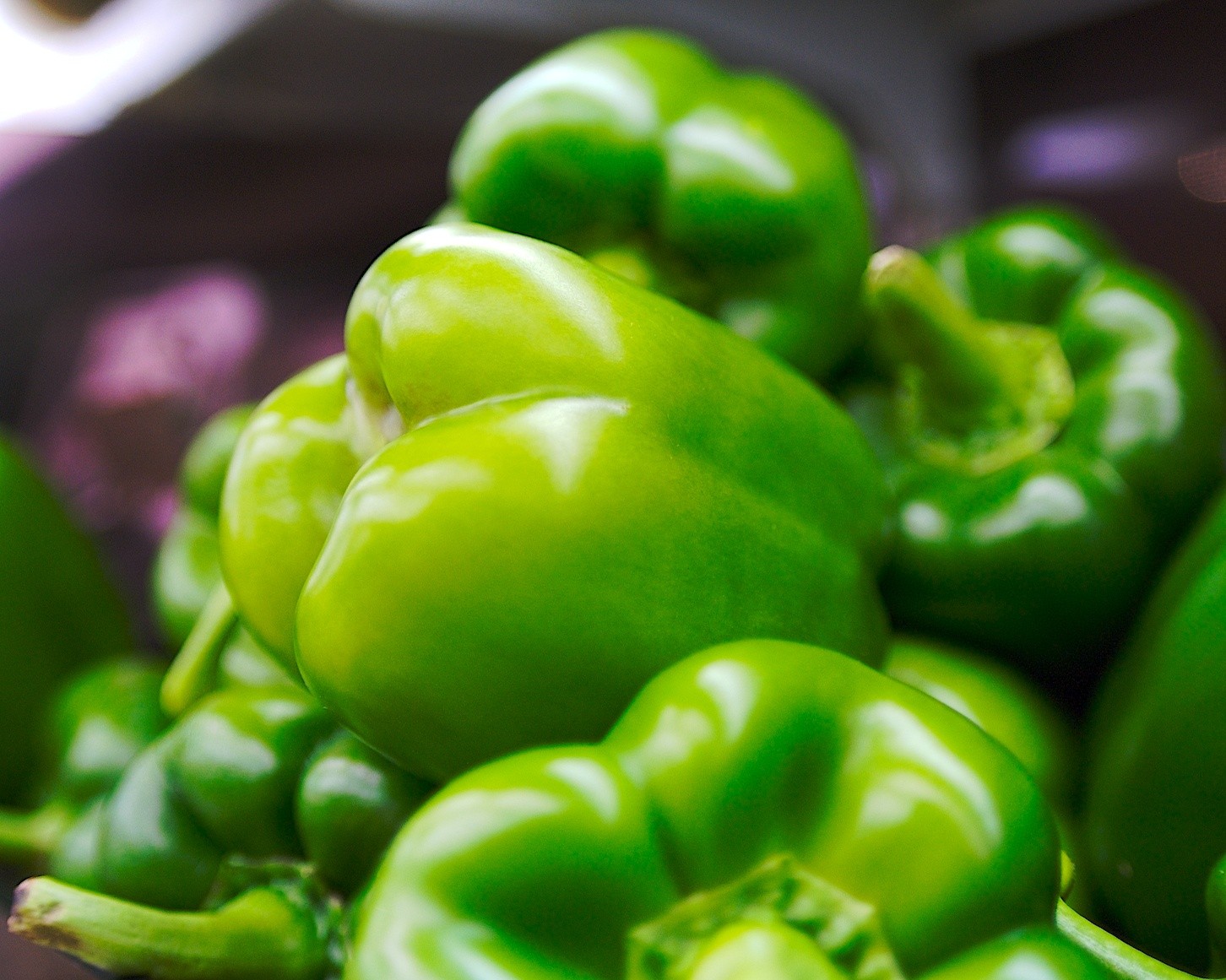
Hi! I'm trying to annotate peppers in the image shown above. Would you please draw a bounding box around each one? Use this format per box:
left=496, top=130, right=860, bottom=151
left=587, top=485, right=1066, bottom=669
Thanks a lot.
left=1, top=29, right=1225, bottom=980
